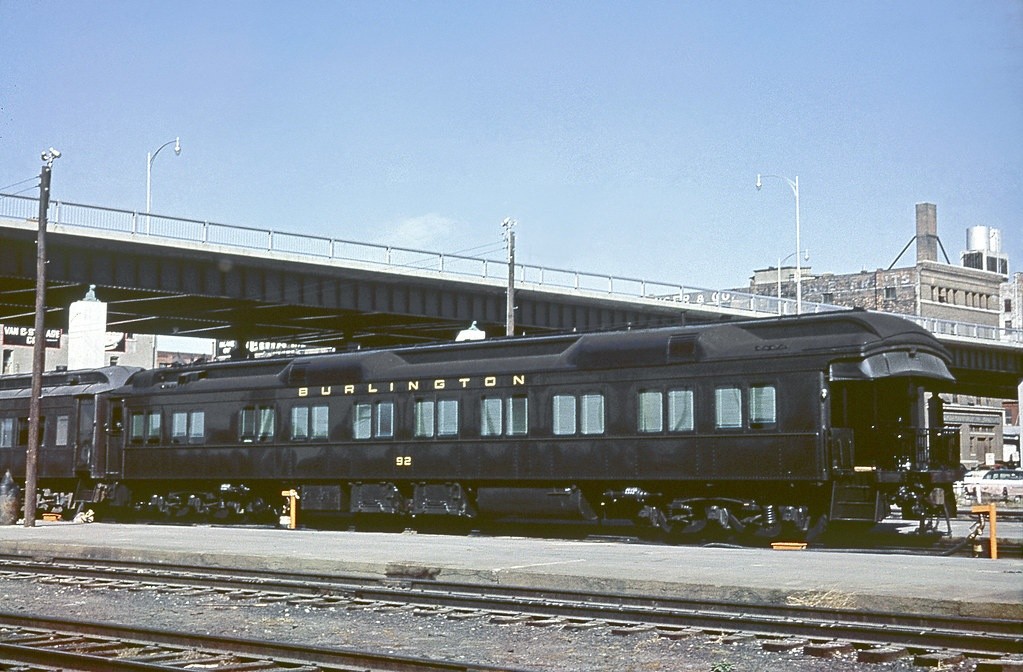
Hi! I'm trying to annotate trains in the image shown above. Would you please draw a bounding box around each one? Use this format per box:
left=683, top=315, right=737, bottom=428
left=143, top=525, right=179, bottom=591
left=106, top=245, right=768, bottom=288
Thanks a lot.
left=1, top=306, right=961, bottom=535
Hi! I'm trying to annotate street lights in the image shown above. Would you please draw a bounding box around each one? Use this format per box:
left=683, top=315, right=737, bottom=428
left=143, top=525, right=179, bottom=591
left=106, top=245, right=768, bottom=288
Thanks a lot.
left=756, top=173, right=803, bottom=316
left=147, top=137, right=182, bottom=235
left=777, top=250, right=809, bottom=317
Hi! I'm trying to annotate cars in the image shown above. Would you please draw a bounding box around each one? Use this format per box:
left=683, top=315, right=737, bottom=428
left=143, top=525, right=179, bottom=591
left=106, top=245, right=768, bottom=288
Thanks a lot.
left=953, top=470, right=1023, bottom=502
